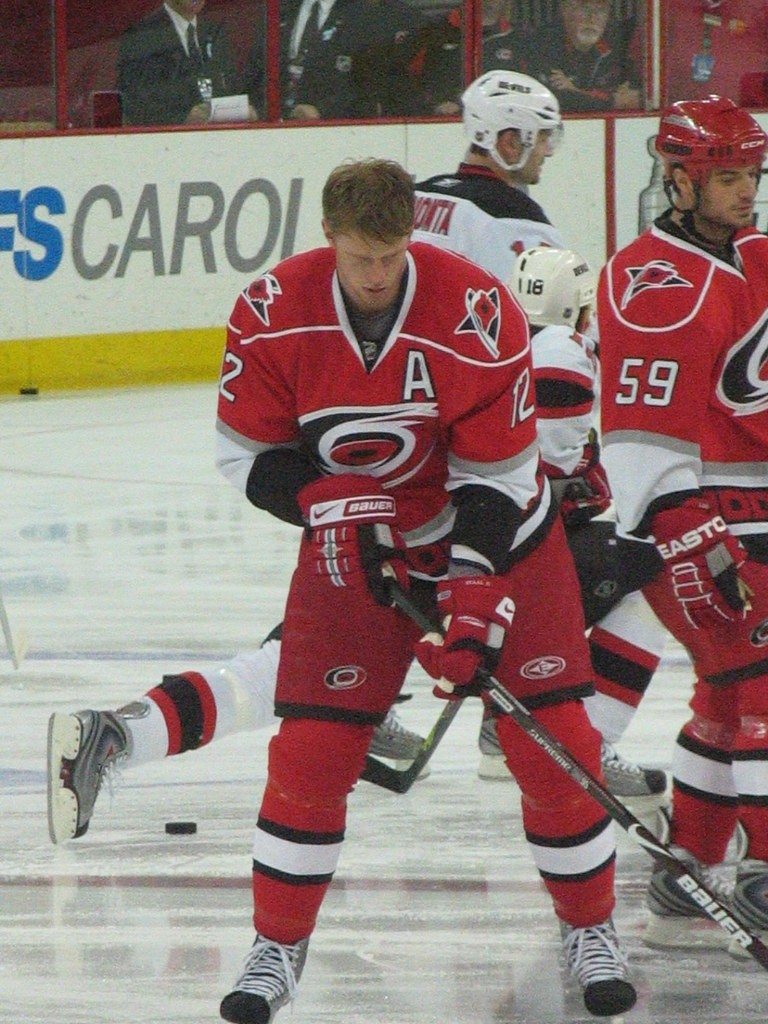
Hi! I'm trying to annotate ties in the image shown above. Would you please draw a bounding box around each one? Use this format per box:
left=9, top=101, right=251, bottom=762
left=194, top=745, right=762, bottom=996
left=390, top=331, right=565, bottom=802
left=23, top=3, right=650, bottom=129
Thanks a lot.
left=186, top=24, right=205, bottom=77
left=297, top=2, right=320, bottom=70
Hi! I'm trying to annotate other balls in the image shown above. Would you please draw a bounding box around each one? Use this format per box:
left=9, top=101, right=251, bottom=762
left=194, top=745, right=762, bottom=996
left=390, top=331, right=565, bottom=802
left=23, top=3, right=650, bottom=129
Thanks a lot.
left=163, top=821, right=198, bottom=835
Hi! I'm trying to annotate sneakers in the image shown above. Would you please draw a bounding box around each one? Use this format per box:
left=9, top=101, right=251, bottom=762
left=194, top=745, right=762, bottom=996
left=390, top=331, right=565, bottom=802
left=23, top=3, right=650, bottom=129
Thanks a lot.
left=478, top=719, right=518, bottom=781
left=220, top=930, right=309, bottom=1024
left=560, top=914, right=637, bottom=1023
left=643, top=846, right=735, bottom=949
left=595, top=738, right=668, bottom=812
left=728, top=872, right=768, bottom=960
left=47, top=701, right=149, bottom=842
left=366, top=717, right=430, bottom=779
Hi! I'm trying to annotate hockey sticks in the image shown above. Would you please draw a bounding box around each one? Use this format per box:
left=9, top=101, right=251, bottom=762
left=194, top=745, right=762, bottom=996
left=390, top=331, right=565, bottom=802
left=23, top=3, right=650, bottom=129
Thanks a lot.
left=385, top=579, right=768, bottom=972
left=359, top=684, right=469, bottom=795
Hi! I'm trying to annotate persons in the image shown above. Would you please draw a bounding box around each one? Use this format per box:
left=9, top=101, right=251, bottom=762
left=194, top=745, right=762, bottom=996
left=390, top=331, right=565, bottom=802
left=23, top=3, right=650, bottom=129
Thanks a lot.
left=46, top=69, right=768, bottom=1024
left=116, top=0, right=640, bottom=126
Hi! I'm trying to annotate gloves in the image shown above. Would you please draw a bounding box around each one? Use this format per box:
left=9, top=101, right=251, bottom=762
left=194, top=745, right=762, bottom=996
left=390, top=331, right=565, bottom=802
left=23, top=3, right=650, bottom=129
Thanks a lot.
left=635, top=489, right=757, bottom=632
left=298, top=475, right=412, bottom=606
left=408, top=576, right=518, bottom=702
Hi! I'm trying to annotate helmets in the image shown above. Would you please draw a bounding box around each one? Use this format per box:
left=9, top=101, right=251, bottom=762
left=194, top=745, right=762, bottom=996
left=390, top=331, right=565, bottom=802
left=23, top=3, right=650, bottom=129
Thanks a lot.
left=509, top=247, right=598, bottom=333
left=462, top=69, right=560, bottom=171
left=657, top=95, right=768, bottom=190
